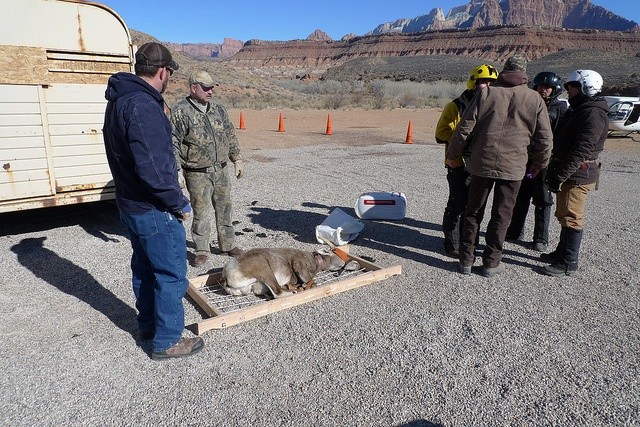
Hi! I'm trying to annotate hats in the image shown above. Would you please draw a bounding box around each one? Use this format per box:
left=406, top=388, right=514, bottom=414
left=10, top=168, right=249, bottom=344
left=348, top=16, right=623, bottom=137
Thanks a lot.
left=134, top=44, right=180, bottom=70
left=189, top=70, right=219, bottom=89
left=504, top=54, right=527, bottom=73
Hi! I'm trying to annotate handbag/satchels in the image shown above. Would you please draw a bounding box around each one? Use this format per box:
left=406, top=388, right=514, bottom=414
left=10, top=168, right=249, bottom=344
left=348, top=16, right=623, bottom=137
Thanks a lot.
left=355, top=192, right=405, bottom=221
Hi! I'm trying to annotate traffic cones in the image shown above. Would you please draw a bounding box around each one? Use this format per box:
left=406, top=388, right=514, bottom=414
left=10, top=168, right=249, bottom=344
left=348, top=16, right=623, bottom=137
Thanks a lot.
left=323, top=113, right=333, bottom=134
left=403, top=119, right=413, bottom=144
left=236, top=111, right=246, bottom=129
left=276, top=112, right=286, bottom=132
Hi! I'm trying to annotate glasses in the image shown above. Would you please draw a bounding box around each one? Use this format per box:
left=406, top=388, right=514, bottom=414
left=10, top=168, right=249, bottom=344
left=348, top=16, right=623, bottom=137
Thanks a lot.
left=162, top=65, right=174, bottom=75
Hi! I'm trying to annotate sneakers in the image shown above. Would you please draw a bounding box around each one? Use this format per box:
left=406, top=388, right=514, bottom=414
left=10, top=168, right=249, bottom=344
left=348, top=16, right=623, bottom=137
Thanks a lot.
left=544, top=266, right=576, bottom=279
left=542, top=251, right=561, bottom=264
left=459, top=262, right=470, bottom=275
left=151, top=336, right=205, bottom=360
left=483, top=264, right=505, bottom=277
left=194, top=255, right=207, bottom=266
left=533, top=239, right=547, bottom=251
left=220, top=247, right=242, bottom=256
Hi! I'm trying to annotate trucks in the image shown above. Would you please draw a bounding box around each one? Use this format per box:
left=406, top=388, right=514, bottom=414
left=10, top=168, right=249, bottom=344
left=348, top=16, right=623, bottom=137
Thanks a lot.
left=0, top=0, right=138, bottom=215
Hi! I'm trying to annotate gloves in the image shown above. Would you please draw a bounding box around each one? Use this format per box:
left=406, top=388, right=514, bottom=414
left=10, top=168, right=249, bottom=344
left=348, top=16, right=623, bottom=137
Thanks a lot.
left=175, top=170, right=186, bottom=188
left=234, top=161, right=246, bottom=179
left=548, top=173, right=562, bottom=196
left=178, top=204, right=191, bottom=220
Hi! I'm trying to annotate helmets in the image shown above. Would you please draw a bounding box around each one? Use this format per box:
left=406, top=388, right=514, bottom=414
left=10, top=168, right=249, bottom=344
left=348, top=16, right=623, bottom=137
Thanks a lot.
left=564, top=70, right=604, bottom=98
left=466, top=65, right=499, bottom=90
left=532, top=72, right=562, bottom=99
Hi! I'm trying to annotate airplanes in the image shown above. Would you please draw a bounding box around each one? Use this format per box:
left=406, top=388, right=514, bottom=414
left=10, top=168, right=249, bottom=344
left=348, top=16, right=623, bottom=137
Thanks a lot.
left=603, top=96, right=640, bottom=137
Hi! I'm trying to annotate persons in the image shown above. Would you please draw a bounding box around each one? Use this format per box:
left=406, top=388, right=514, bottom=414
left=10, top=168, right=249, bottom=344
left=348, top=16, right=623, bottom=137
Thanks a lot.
left=101, top=40, right=204, bottom=361
left=434, top=63, right=501, bottom=258
left=505, top=72, right=568, bottom=252
left=539, top=69, right=610, bottom=277
left=168, top=69, right=249, bottom=268
left=443, top=55, right=555, bottom=278
